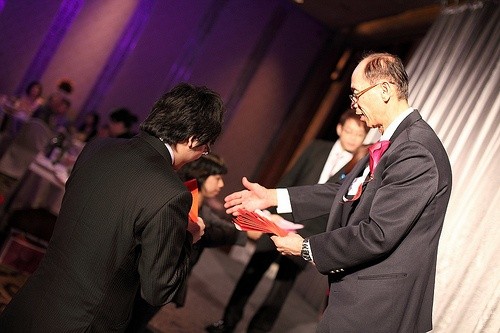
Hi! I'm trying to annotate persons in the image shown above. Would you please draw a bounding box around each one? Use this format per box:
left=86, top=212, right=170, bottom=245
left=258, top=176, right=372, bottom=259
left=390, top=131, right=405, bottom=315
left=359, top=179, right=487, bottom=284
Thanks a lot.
left=223, top=53, right=452, bottom=333
left=0, top=81, right=223, bottom=333
left=125, top=154, right=228, bottom=333
left=204, top=109, right=370, bottom=333
left=0, top=81, right=140, bottom=139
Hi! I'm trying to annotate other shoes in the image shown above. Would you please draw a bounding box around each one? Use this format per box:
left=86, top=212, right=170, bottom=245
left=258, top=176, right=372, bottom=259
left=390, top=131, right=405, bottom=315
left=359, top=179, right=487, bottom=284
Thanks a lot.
left=204, top=319, right=225, bottom=333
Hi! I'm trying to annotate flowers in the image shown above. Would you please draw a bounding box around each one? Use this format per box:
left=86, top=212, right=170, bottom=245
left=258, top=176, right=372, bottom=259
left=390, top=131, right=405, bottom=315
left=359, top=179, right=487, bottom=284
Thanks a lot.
left=364, top=139, right=392, bottom=185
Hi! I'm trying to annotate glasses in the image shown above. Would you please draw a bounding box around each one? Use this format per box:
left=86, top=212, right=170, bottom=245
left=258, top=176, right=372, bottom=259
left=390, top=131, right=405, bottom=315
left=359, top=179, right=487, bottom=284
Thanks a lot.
left=348, top=82, right=380, bottom=104
left=202, top=142, right=211, bottom=156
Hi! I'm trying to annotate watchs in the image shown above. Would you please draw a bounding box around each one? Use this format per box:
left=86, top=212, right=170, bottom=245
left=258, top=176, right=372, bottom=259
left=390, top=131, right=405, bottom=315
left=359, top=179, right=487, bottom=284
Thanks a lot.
left=301, top=238, right=311, bottom=261
